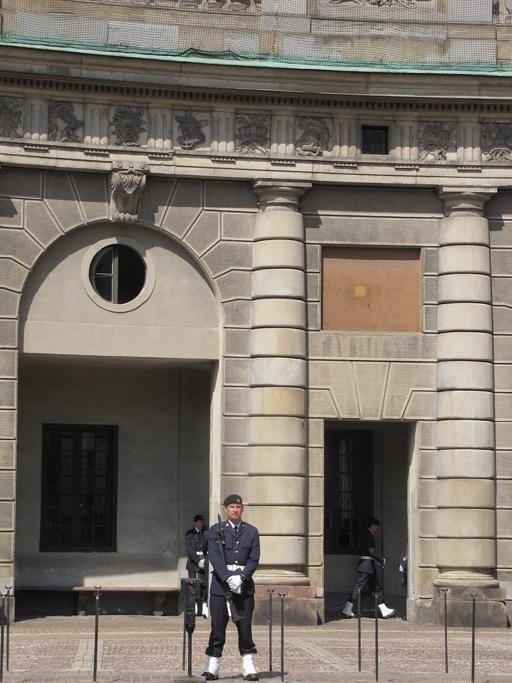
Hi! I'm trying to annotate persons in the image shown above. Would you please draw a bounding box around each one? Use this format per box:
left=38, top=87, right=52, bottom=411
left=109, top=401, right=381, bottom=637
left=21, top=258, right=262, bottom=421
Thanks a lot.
left=342, top=517, right=398, bottom=619
left=185, top=514, right=208, bottom=614
left=397, top=539, right=407, bottom=620
left=201, top=494, right=260, bottom=680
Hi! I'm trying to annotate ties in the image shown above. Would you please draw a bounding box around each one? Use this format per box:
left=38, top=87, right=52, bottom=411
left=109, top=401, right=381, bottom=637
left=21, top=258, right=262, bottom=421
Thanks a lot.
left=234, top=526, right=238, bottom=535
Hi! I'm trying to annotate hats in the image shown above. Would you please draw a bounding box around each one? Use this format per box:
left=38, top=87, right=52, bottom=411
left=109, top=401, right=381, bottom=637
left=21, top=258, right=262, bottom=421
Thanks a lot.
left=224, top=494, right=242, bottom=508
left=194, top=515, right=202, bottom=522
left=374, top=556, right=388, bottom=569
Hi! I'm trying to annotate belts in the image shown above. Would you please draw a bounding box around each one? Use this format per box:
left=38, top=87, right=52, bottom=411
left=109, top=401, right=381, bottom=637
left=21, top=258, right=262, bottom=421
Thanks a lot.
left=359, top=556, right=376, bottom=560
left=195, top=551, right=208, bottom=556
left=226, top=564, right=246, bottom=572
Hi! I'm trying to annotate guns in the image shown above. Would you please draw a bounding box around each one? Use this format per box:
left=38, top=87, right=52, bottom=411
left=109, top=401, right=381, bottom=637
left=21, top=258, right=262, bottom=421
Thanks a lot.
left=216, top=513, right=245, bottom=624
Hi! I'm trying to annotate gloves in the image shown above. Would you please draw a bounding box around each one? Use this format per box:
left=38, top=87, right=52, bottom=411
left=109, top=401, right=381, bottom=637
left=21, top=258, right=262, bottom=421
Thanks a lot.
left=198, top=559, right=205, bottom=569
left=226, top=575, right=244, bottom=596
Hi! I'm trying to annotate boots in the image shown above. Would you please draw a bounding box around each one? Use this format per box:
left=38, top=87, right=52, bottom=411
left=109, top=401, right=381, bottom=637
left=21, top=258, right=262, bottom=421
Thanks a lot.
left=202, top=602, right=208, bottom=619
left=195, top=602, right=199, bottom=616
left=201, top=656, right=220, bottom=680
left=377, top=602, right=400, bottom=619
left=241, top=653, right=259, bottom=681
left=341, top=600, right=357, bottom=618
